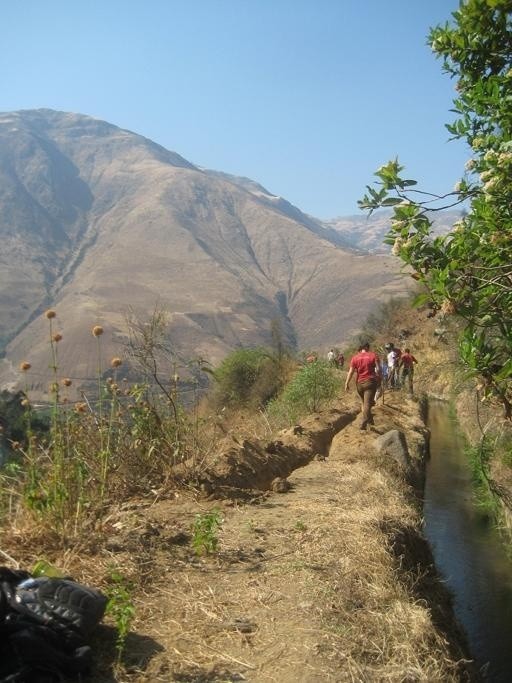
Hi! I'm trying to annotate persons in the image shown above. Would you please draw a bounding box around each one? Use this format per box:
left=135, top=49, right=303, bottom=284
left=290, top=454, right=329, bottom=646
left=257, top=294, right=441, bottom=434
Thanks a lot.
left=326, top=347, right=338, bottom=369
left=398, top=346, right=419, bottom=393
left=384, top=342, right=398, bottom=390
left=392, top=342, right=402, bottom=386
left=344, top=340, right=381, bottom=430
left=375, top=361, right=384, bottom=400
left=335, top=351, right=345, bottom=366
